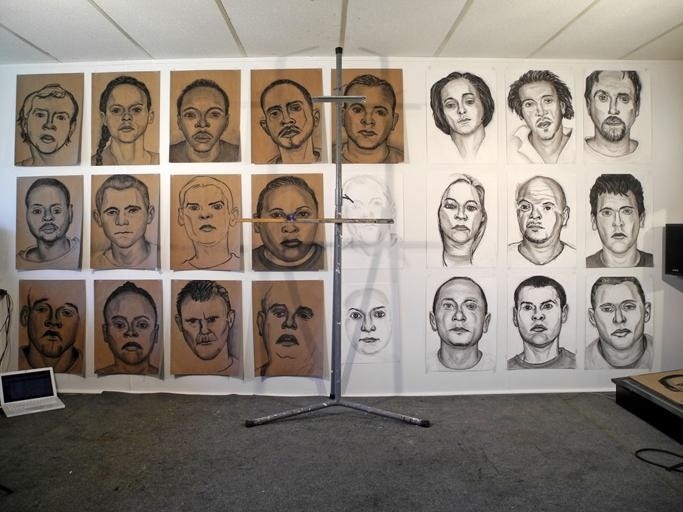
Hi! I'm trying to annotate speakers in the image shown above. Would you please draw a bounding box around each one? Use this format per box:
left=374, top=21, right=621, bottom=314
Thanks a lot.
left=664, top=223, right=683, bottom=277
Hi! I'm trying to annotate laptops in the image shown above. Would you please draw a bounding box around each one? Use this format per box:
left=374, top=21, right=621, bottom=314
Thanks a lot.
left=0, top=367, right=66, bottom=418
